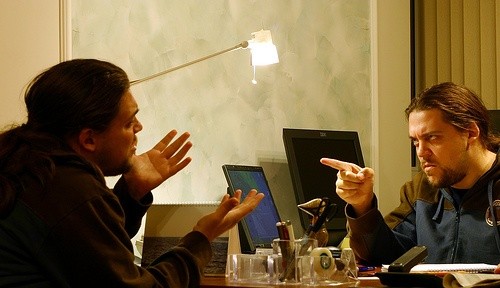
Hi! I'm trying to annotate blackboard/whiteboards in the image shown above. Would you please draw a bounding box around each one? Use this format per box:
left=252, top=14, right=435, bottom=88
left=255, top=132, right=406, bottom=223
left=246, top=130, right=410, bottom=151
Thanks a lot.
left=59, top=0, right=381, bottom=216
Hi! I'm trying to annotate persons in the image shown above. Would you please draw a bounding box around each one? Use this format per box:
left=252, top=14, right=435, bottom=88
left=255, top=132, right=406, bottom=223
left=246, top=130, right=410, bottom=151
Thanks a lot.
left=320, top=80, right=500, bottom=275
left=0, top=60, right=269, bottom=288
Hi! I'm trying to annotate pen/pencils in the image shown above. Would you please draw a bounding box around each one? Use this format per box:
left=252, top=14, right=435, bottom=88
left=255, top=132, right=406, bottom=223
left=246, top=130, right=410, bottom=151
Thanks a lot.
left=275, top=217, right=300, bottom=283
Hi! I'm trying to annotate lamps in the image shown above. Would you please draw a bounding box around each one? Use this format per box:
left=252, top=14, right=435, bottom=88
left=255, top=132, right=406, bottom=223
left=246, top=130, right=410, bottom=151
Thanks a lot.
left=125, top=29, right=279, bottom=89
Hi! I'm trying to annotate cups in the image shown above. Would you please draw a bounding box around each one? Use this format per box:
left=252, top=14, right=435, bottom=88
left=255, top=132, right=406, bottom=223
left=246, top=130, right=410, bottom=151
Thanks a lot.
left=295, top=237, right=319, bottom=286
left=272, top=238, right=296, bottom=285
left=226, top=251, right=282, bottom=284
left=297, top=255, right=333, bottom=288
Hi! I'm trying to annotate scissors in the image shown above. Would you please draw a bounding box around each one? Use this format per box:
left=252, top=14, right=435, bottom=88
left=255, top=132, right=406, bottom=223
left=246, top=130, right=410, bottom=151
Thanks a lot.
left=286, top=196, right=338, bottom=279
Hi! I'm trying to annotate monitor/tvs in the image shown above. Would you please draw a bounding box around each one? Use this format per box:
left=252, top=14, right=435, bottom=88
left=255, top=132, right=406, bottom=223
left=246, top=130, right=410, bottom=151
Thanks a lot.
left=222, top=164, right=283, bottom=254
left=282, top=127, right=366, bottom=230
